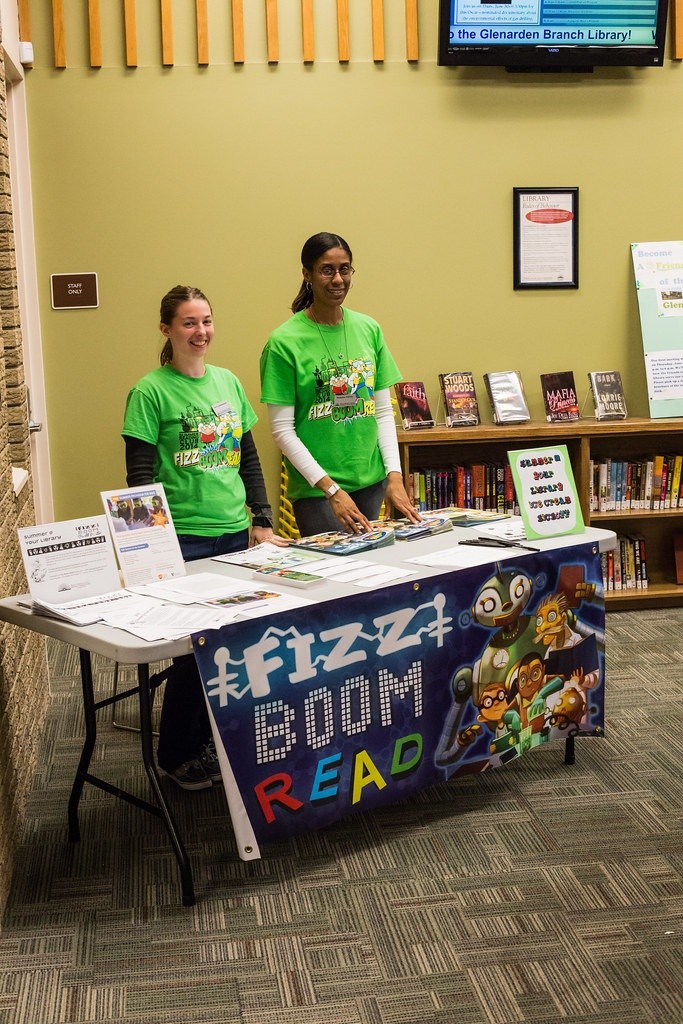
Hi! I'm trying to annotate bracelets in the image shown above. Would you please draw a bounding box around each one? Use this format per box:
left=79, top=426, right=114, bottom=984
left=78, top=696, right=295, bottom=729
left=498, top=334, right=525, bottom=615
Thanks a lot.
left=326, top=483, right=341, bottom=499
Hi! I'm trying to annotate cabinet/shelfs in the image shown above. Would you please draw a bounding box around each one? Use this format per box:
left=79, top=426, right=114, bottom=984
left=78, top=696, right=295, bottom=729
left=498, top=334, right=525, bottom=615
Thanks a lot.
left=396, top=416, right=683, bottom=601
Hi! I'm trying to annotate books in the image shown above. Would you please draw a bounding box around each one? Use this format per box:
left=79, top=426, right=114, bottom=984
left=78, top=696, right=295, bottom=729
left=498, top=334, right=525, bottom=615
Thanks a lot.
left=540, top=372, right=580, bottom=423
left=394, top=381, right=434, bottom=430
left=408, top=463, right=522, bottom=517
left=588, top=454, right=683, bottom=512
left=440, top=372, right=481, bottom=426
left=598, top=531, right=648, bottom=590
left=483, top=371, right=531, bottom=423
left=589, top=371, right=628, bottom=421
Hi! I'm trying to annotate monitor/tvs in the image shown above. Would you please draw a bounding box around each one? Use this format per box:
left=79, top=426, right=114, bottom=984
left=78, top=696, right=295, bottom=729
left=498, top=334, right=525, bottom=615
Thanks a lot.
left=436, top=0, right=668, bottom=74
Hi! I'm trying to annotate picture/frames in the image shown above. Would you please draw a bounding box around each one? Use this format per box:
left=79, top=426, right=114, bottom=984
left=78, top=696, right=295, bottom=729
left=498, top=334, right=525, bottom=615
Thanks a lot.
left=512, top=189, right=581, bottom=290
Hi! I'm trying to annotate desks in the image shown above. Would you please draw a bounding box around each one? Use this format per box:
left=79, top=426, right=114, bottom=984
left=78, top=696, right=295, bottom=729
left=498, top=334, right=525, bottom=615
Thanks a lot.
left=0, top=508, right=614, bottom=906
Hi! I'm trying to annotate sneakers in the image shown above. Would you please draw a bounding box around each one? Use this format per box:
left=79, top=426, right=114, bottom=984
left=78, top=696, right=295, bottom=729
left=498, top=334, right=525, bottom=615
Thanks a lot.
left=165, top=758, right=212, bottom=790
left=198, top=750, right=222, bottom=781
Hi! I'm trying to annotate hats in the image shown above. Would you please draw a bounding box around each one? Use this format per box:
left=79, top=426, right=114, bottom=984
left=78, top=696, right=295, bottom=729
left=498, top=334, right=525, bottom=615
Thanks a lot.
left=153, top=496, right=162, bottom=507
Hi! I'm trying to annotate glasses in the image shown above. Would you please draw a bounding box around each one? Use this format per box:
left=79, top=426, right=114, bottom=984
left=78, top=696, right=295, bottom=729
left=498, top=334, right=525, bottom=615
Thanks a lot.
left=312, top=266, right=355, bottom=277
left=152, top=502, right=159, bottom=505
left=119, top=504, right=126, bottom=508
left=134, top=504, right=142, bottom=507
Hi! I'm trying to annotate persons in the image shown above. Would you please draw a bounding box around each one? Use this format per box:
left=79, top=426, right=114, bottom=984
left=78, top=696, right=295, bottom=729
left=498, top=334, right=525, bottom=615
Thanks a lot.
left=121, top=285, right=295, bottom=791
left=107, top=496, right=169, bottom=526
left=260, top=231, right=421, bottom=536
